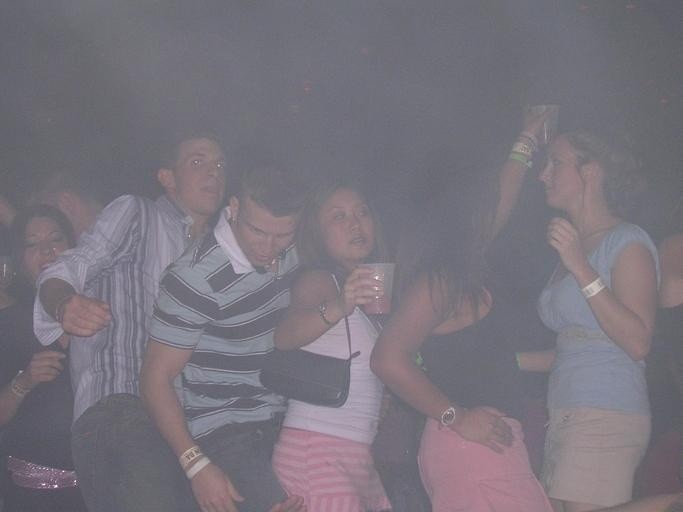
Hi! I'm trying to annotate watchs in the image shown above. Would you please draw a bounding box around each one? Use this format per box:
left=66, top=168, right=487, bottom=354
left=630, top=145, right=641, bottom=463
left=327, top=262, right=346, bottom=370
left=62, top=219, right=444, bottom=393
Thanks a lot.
left=438, top=403, right=459, bottom=425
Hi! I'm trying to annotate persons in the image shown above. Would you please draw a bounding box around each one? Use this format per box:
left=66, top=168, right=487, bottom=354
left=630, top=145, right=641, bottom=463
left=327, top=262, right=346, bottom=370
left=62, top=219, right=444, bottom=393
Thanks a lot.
left=368, top=103, right=554, bottom=511
left=512, top=131, right=683, bottom=510
left=138, top=170, right=299, bottom=510
left=32, top=132, right=231, bottom=510
left=274, top=178, right=393, bottom=512
left=0, top=179, right=103, bottom=511
left=639, top=220, right=682, bottom=492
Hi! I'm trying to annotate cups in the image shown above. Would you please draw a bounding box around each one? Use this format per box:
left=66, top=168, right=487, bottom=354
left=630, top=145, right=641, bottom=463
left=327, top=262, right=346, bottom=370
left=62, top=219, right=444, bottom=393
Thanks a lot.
left=529, top=103, right=561, bottom=147
left=355, top=261, right=397, bottom=317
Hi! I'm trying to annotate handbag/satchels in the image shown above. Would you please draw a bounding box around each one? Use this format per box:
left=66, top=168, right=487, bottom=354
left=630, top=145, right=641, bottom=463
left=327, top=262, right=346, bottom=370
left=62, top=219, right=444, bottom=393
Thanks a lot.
left=260, top=346, right=349, bottom=408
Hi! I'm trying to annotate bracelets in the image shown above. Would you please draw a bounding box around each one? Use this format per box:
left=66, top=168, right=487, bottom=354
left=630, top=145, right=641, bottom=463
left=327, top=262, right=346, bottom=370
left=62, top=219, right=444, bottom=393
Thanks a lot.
left=186, top=454, right=210, bottom=479
left=318, top=302, right=333, bottom=324
left=177, top=443, right=201, bottom=468
left=582, top=276, right=605, bottom=299
left=511, top=131, right=537, bottom=164
